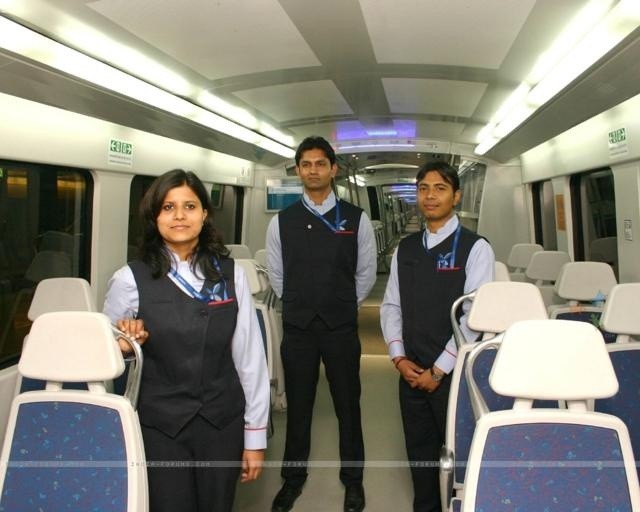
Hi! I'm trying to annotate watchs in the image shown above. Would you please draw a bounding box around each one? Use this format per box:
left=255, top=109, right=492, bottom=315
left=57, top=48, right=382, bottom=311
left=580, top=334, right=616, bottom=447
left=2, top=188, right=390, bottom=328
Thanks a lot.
left=429, top=366, right=445, bottom=382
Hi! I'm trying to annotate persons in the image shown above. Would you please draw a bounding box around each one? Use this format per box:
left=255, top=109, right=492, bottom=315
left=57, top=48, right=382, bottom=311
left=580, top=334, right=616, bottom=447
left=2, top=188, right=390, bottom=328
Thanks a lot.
left=266, top=136, right=378, bottom=511
left=376, top=160, right=499, bottom=510
left=98, top=170, right=273, bottom=512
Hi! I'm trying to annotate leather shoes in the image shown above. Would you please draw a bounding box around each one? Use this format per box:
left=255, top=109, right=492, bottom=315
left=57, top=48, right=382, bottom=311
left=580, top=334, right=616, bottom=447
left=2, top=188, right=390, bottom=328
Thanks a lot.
left=344, top=485, right=365, bottom=512
left=271, top=481, right=302, bottom=512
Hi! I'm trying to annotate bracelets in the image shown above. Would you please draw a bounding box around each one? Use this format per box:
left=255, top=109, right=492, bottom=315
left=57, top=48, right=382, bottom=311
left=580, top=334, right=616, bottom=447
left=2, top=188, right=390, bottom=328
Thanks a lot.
left=396, top=358, right=407, bottom=368
